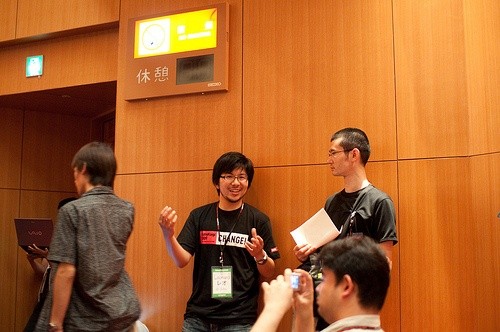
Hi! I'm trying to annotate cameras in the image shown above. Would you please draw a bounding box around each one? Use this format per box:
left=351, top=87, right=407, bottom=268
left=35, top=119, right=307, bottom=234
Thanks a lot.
left=290, top=273, right=302, bottom=292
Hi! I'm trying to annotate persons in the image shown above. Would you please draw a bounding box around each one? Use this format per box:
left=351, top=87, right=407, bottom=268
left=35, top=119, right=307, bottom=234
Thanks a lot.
left=248, top=236, right=391, bottom=332
left=22, top=197, right=79, bottom=332
left=292, top=126, right=399, bottom=332
left=47, top=140, right=143, bottom=332
left=159, top=152, right=281, bottom=332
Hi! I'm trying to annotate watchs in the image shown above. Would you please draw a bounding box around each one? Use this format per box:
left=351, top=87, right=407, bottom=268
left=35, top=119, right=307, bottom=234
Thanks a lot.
left=48, top=322, right=63, bottom=332
left=253, top=250, right=268, bottom=264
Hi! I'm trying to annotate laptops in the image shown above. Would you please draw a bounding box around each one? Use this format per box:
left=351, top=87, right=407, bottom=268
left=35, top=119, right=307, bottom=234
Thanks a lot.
left=14, top=218, right=54, bottom=255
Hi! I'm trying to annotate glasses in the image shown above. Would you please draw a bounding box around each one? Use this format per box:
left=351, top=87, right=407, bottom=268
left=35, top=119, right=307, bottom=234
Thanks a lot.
left=328, top=148, right=357, bottom=158
left=218, top=173, right=249, bottom=183
